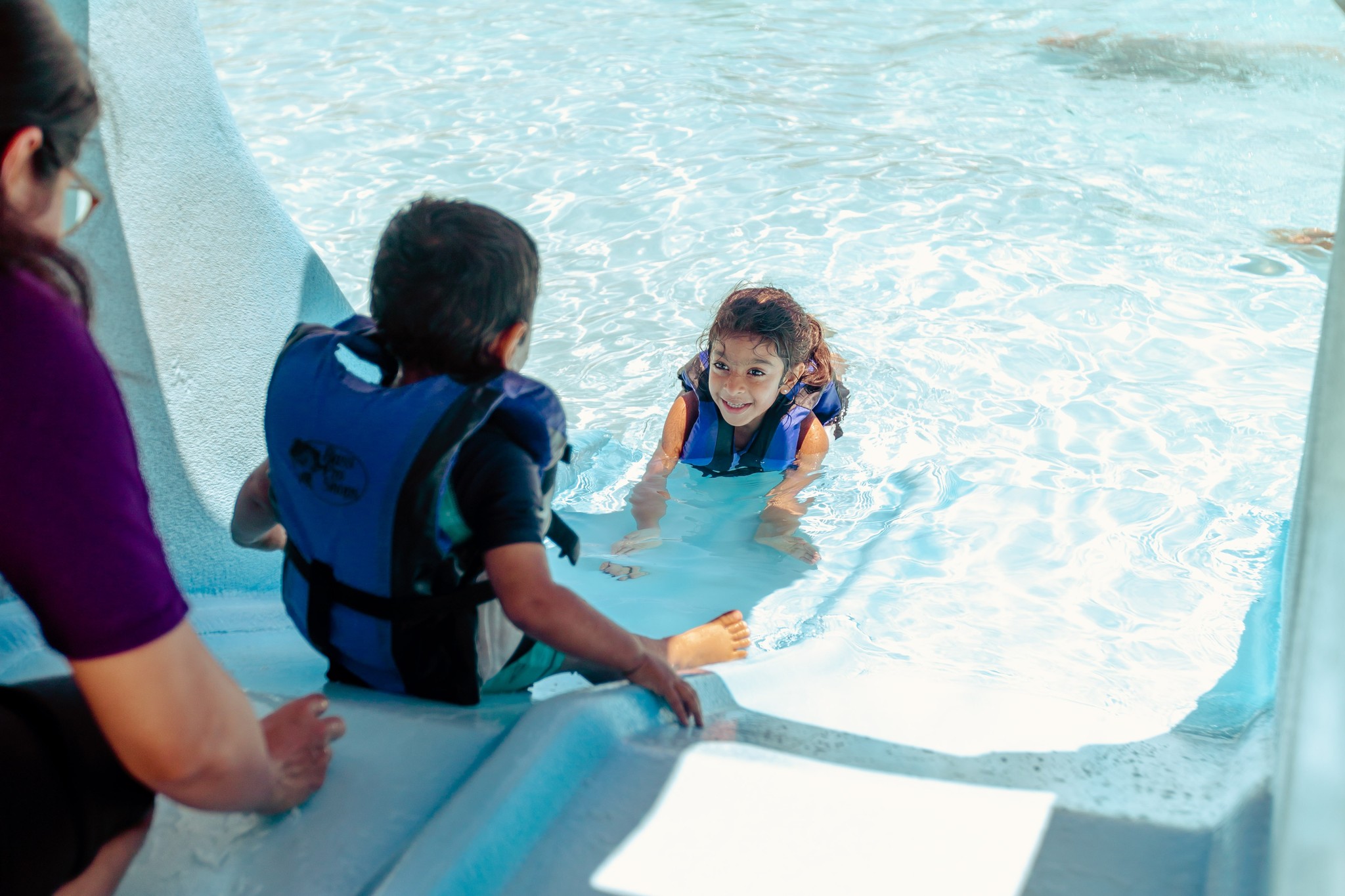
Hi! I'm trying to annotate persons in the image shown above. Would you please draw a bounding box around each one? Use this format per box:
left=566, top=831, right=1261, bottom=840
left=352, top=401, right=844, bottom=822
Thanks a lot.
left=230, top=195, right=750, bottom=727
left=0, top=0, right=347, bottom=894
left=600, top=278, right=847, bottom=582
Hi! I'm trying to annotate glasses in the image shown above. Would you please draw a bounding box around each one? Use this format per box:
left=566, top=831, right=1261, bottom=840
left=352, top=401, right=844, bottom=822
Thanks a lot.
left=33, top=145, right=104, bottom=238
